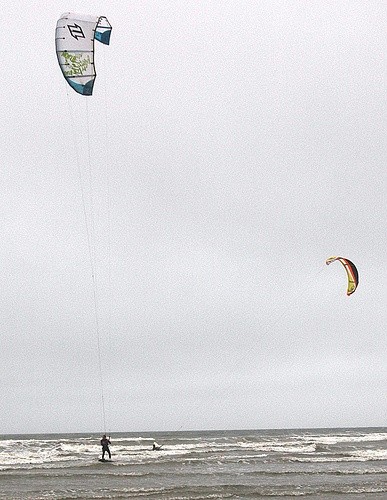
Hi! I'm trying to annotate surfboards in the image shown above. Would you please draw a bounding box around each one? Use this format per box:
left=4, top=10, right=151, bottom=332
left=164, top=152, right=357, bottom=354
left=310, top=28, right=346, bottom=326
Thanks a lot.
left=97, top=458, right=116, bottom=463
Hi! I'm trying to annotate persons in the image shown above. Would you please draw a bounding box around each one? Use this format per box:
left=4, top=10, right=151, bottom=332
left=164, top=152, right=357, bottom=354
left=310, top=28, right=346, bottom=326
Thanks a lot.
left=152, top=444, right=160, bottom=451
left=100, top=435, right=112, bottom=461
